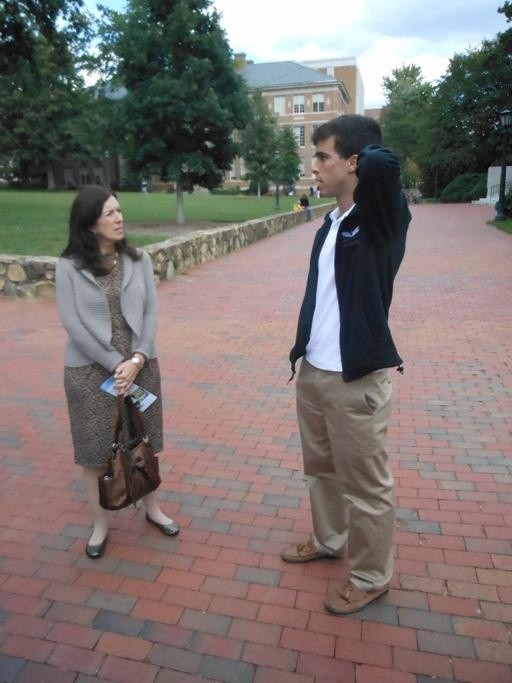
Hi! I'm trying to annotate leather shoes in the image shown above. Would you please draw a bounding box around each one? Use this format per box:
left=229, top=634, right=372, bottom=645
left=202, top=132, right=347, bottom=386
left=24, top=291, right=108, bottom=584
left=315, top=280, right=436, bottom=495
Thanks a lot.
left=281, top=535, right=345, bottom=563
left=324, top=577, right=389, bottom=615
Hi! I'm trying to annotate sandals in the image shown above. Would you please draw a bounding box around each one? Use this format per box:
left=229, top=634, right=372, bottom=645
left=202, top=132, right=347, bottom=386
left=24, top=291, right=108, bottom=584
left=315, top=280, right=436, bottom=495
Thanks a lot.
left=145, top=512, right=179, bottom=536
left=86, top=529, right=108, bottom=558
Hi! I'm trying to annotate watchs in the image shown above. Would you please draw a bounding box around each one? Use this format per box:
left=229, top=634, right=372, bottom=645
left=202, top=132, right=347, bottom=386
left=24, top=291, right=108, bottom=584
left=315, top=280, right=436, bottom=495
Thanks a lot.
left=129, top=355, right=143, bottom=371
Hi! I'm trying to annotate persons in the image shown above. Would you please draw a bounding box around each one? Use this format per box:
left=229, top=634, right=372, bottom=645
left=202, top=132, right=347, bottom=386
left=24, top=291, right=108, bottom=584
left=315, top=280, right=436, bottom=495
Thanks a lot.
left=281, top=114, right=412, bottom=616
left=293, top=195, right=313, bottom=222
left=141, top=175, right=148, bottom=196
left=55, top=185, right=179, bottom=559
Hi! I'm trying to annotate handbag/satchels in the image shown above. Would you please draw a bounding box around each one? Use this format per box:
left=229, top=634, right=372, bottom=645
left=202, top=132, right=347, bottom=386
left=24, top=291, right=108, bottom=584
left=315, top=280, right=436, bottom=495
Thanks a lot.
left=98, top=393, right=161, bottom=510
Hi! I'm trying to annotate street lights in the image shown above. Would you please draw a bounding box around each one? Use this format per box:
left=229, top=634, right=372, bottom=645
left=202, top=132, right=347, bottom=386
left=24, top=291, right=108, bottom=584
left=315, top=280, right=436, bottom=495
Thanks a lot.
left=495, top=107, right=511, bottom=221
